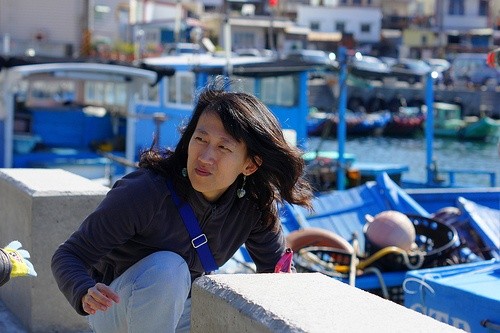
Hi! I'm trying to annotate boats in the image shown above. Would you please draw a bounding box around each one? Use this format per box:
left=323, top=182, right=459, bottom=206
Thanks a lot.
left=0, top=12, right=500, bottom=332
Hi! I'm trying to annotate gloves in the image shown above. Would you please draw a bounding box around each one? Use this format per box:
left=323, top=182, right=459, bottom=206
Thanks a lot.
left=2, top=240, right=37, bottom=278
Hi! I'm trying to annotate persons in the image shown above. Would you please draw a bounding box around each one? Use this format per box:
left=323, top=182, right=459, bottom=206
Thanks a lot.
left=51, top=73, right=316, bottom=333
left=0, top=240, right=37, bottom=287
left=363, top=208, right=419, bottom=269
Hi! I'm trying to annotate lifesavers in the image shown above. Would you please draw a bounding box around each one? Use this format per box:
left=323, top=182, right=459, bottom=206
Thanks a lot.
left=284, top=230, right=358, bottom=262
